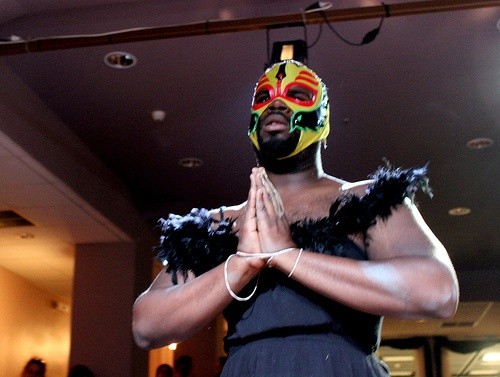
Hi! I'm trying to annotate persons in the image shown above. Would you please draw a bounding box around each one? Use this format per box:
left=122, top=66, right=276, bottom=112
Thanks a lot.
left=69, top=353, right=229, bottom=376
left=131, top=59, right=460, bottom=376
left=20, top=357, right=47, bottom=377
